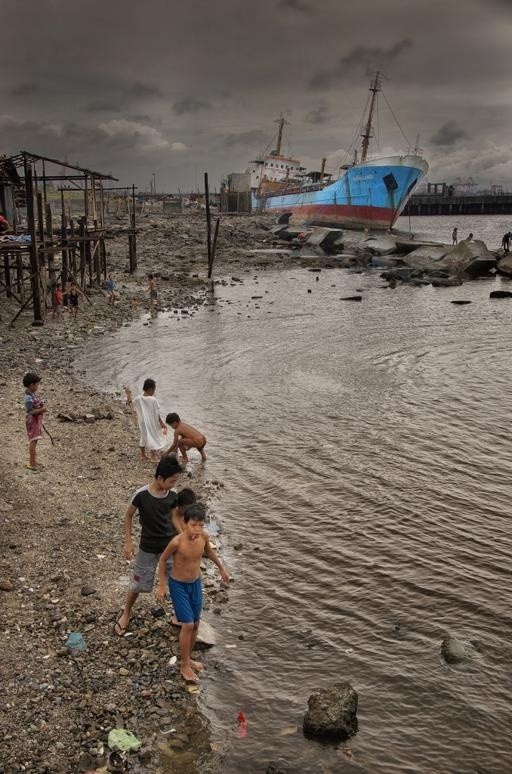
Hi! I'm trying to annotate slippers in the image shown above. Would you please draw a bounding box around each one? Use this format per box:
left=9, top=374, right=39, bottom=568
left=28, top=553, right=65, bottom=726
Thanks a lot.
left=113, top=615, right=127, bottom=636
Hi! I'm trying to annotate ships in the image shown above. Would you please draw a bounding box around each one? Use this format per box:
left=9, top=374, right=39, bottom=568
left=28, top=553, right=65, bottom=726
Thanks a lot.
left=219, top=67, right=431, bottom=231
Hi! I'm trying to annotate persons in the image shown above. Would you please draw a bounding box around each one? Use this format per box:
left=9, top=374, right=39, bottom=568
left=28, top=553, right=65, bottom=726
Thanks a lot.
left=1, top=213, right=10, bottom=230
left=115, top=455, right=182, bottom=636
left=62, top=282, right=77, bottom=308
left=145, top=276, right=157, bottom=303
left=165, top=413, right=208, bottom=462
left=170, top=490, right=196, bottom=535
left=501, top=230, right=511, bottom=250
left=22, top=372, right=50, bottom=471
left=123, top=379, right=168, bottom=462
left=55, top=284, right=66, bottom=318
left=451, top=228, right=458, bottom=245
left=157, top=505, right=230, bottom=682
left=467, top=233, right=472, bottom=240
left=105, top=273, right=115, bottom=306
